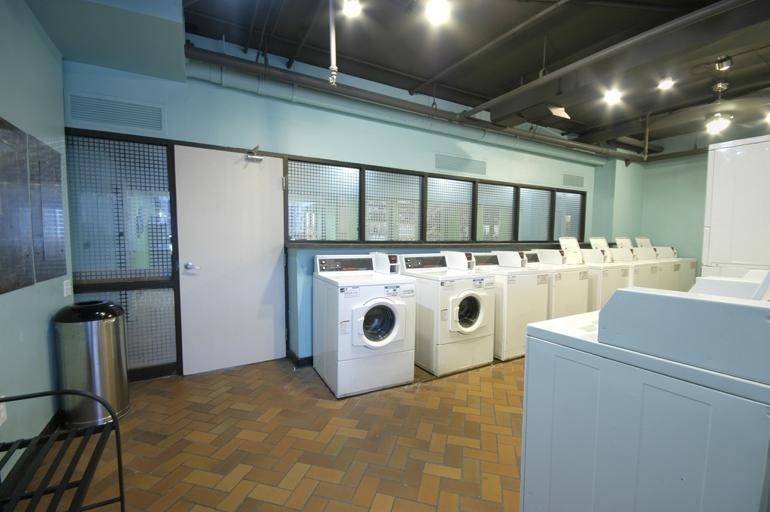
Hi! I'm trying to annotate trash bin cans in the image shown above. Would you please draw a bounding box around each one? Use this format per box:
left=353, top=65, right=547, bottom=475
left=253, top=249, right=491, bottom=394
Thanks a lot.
left=49, top=299, right=132, bottom=431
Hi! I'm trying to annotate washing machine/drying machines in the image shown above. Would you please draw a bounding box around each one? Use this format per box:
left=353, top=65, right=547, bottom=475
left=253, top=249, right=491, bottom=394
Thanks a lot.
left=313, top=253, right=417, bottom=400
left=471, top=252, right=548, bottom=362
left=399, top=254, right=495, bottom=378
left=533, top=235, right=693, bottom=323
